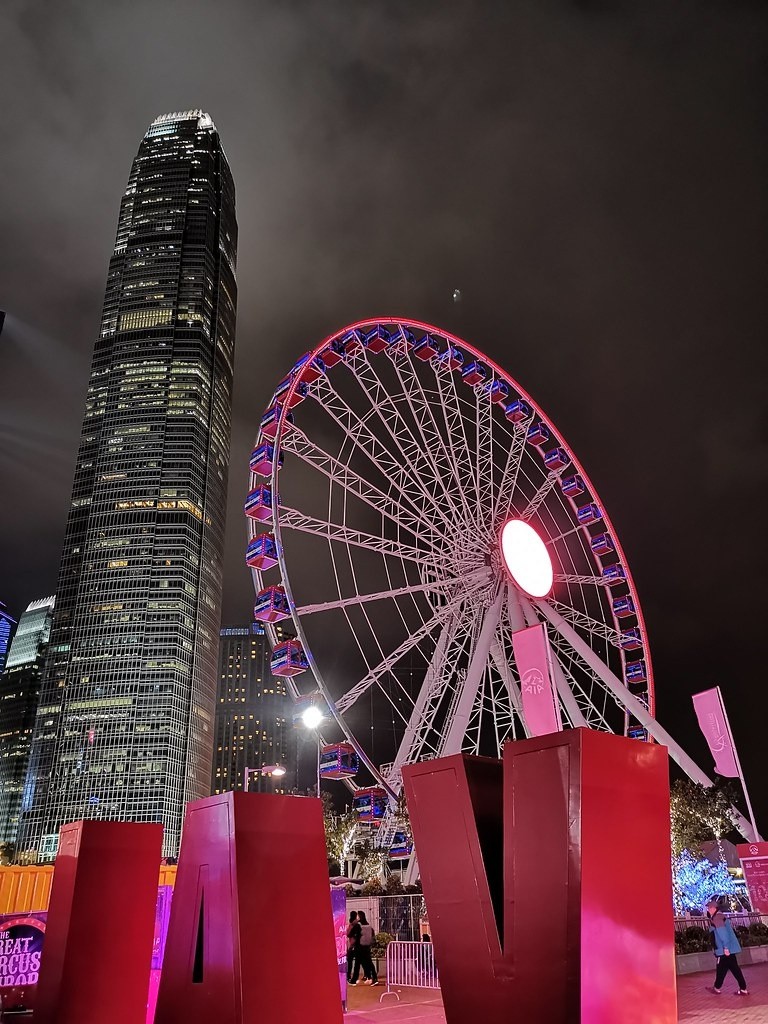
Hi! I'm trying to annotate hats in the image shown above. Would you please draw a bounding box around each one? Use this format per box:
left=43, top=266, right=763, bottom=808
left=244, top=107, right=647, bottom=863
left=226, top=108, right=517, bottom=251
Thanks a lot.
left=705, top=900, right=719, bottom=908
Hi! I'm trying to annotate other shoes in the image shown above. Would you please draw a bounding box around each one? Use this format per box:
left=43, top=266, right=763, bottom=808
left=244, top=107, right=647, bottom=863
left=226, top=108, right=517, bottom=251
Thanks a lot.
left=371, top=981, right=378, bottom=986
left=348, top=982, right=356, bottom=986
left=364, top=979, right=372, bottom=984
left=349, top=979, right=359, bottom=983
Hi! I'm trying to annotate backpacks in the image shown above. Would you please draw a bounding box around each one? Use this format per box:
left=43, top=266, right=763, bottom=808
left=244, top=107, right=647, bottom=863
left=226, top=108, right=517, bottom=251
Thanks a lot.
left=358, top=921, right=372, bottom=945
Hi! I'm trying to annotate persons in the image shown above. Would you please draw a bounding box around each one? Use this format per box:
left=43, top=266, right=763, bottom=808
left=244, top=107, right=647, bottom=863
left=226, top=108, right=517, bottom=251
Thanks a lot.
left=705, top=901, right=750, bottom=996
left=346, top=911, right=379, bottom=986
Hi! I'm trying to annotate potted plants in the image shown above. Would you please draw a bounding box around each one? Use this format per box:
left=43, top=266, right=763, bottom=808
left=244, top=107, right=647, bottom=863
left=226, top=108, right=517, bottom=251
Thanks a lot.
left=674, top=921, right=768, bottom=975
left=350, top=933, right=405, bottom=977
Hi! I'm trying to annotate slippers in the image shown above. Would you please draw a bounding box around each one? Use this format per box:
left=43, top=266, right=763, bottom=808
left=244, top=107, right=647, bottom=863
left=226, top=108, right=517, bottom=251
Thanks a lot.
left=705, top=986, right=720, bottom=994
left=734, top=990, right=749, bottom=995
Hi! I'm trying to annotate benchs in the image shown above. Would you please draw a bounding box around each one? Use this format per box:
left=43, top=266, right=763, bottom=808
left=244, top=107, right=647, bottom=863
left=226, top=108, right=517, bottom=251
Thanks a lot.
left=406, top=958, right=439, bottom=978
